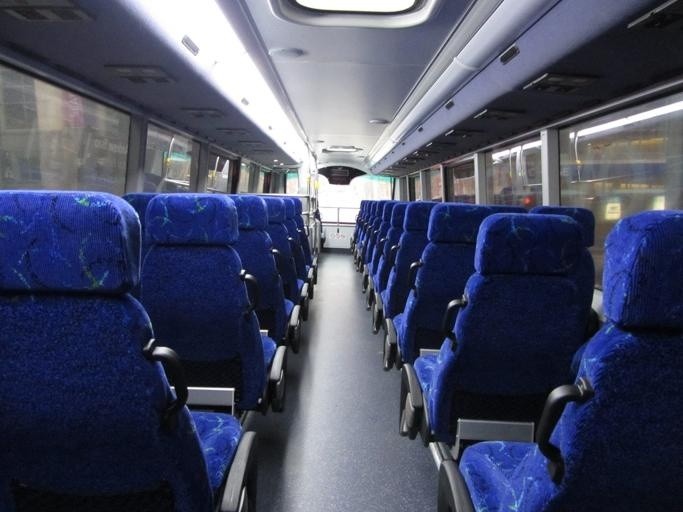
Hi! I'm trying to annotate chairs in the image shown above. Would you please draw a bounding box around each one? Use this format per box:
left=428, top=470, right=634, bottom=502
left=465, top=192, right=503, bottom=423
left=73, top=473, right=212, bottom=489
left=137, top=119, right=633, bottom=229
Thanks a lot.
left=347, top=198, right=683, bottom=511
left=3, top=187, right=326, bottom=511
